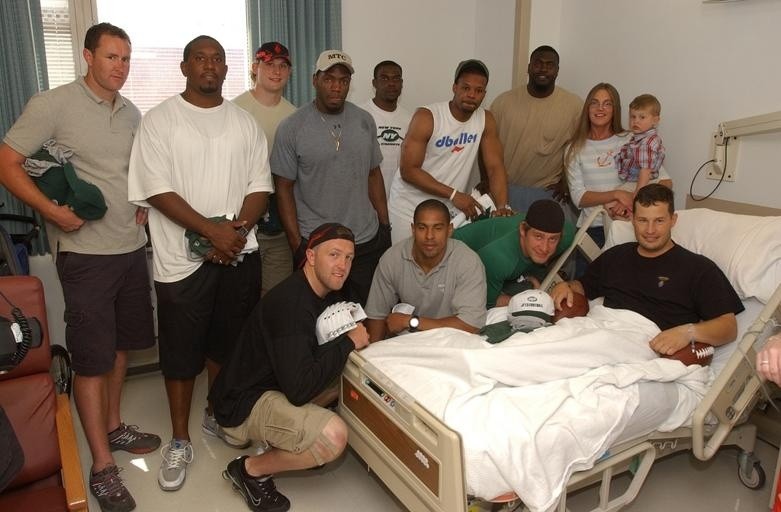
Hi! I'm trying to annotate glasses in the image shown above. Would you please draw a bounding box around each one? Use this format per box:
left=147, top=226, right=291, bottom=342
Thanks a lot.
left=590, top=102, right=613, bottom=109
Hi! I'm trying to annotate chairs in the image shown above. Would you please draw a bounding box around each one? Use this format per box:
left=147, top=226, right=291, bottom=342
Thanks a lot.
left=0, top=274, right=88, bottom=512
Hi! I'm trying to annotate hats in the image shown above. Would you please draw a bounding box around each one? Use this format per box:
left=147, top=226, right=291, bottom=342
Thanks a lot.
left=525, top=199, right=565, bottom=233
left=297, top=222, right=356, bottom=269
left=509, top=289, right=555, bottom=322
left=63, top=161, right=108, bottom=222
left=255, top=42, right=292, bottom=68
left=313, top=49, right=355, bottom=76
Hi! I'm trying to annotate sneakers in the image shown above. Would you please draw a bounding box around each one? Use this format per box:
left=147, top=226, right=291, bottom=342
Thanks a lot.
left=221, top=455, right=291, bottom=512
left=107, top=423, right=162, bottom=455
left=157, top=438, right=192, bottom=492
left=201, top=408, right=252, bottom=449
left=89, top=464, right=136, bottom=512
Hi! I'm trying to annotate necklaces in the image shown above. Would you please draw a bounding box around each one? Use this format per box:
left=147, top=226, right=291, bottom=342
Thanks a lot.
left=373, top=98, right=396, bottom=109
left=316, top=106, right=346, bottom=151
left=451, top=106, right=471, bottom=121
left=413, top=244, right=444, bottom=266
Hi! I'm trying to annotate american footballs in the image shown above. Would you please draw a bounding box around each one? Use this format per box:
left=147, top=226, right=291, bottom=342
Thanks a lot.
left=553, top=292, right=589, bottom=322
left=661, top=341, right=714, bottom=365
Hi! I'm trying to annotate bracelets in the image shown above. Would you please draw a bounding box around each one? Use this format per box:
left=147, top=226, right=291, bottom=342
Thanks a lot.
left=499, top=204, right=512, bottom=210
left=449, top=188, right=457, bottom=200
left=687, top=321, right=698, bottom=342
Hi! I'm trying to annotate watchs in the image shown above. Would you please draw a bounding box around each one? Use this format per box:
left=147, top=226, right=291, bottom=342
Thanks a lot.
left=408, top=315, right=419, bottom=332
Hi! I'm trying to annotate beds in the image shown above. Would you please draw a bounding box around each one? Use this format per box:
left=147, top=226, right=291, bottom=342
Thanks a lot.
left=336, top=196, right=781, bottom=512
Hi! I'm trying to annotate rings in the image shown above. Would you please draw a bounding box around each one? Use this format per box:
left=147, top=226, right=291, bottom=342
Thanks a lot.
left=219, top=260, right=223, bottom=264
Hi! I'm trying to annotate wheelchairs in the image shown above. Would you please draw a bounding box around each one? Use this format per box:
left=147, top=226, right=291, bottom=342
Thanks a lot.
left=0, top=199, right=71, bottom=403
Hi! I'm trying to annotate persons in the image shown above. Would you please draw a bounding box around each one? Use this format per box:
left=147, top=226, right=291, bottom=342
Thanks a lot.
left=268, top=49, right=393, bottom=302
left=562, top=82, right=673, bottom=278
left=487, top=43, right=588, bottom=208
left=358, top=61, right=411, bottom=222
left=391, top=58, right=512, bottom=247
left=362, top=198, right=488, bottom=343
left=548, top=185, right=745, bottom=355
left=603, top=90, right=665, bottom=220
left=208, top=222, right=373, bottom=511
left=0, top=22, right=162, bottom=510
left=451, top=199, right=578, bottom=306
left=232, top=37, right=300, bottom=298
left=129, top=32, right=275, bottom=490
